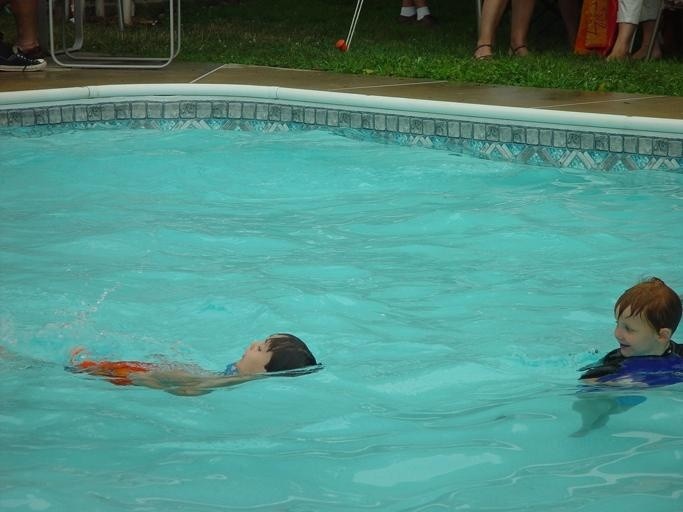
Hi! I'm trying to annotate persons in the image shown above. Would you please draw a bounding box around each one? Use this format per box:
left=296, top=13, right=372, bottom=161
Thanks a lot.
left=62, top=331, right=315, bottom=396
left=559, top=0, right=579, bottom=49
left=602, top=0, right=665, bottom=68
left=574, top=277, right=683, bottom=393
left=398, top=0, right=437, bottom=28
left=5, top=0, right=41, bottom=55
left=472, top=0, right=535, bottom=64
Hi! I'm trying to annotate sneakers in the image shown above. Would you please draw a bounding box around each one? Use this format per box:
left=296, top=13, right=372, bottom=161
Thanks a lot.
left=0, top=45, right=49, bottom=72
left=396, top=13, right=440, bottom=30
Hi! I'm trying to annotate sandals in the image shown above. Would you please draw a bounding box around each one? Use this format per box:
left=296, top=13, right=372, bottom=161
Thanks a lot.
left=473, top=44, right=492, bottom=62
